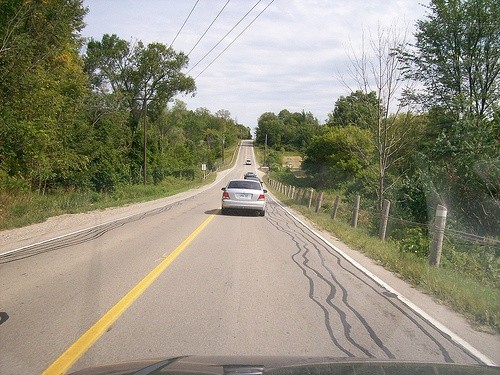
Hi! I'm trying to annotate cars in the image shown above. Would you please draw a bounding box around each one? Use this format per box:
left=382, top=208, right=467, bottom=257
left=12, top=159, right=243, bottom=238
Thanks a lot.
left=244, top=171, right=260, bottom=181
left=246, top=159, right=252, bottom=165
left=220, top=180, right=267, bottom=216
